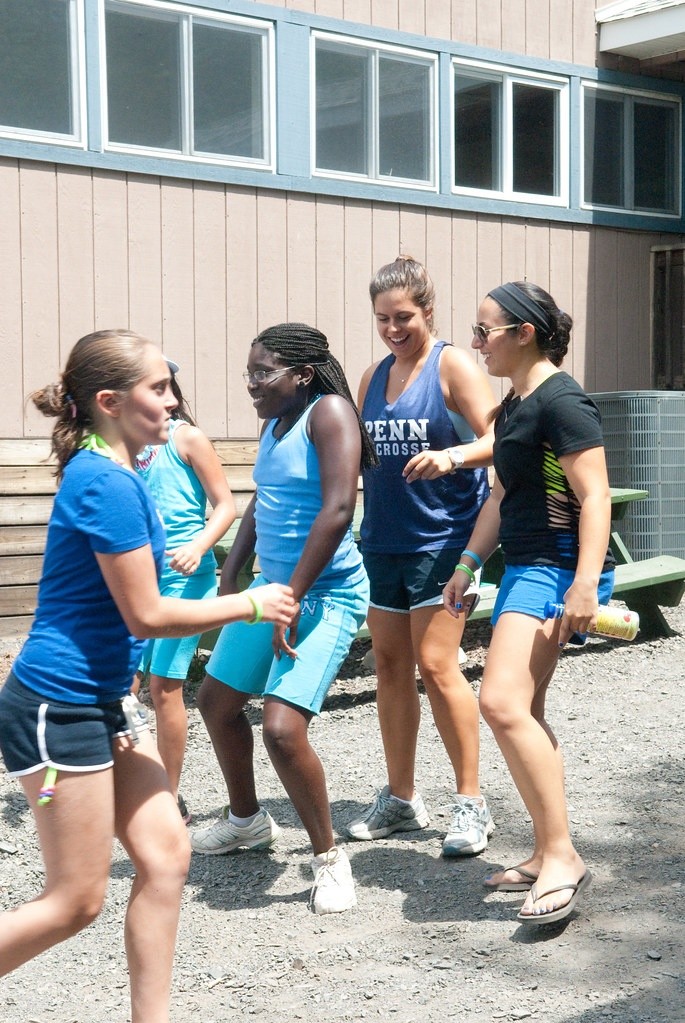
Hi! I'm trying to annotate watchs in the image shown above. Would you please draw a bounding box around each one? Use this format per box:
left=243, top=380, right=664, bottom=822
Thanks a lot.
left=445, top=447, right=464, bottom=475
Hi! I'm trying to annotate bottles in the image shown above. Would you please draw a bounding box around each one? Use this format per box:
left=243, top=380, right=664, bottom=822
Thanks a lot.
left=543, top=599, right=640, bottom=642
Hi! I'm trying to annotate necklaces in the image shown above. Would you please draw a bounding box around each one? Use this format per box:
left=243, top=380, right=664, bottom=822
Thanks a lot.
left=394, top=369, right=404, bottom=382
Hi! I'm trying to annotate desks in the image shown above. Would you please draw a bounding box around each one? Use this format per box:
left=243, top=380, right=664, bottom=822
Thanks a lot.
left=212, top=488, right=684, bottom=645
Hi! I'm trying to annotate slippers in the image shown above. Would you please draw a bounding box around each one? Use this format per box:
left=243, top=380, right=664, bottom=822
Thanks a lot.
left=485, top=865, right=538, bottom=893
left=516, top=867, right=593, bottom=925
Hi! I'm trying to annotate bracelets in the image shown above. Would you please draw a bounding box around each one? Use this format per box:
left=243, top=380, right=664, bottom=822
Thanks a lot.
left=461, top=550, right=482, bottom=567
left=247, top=592, right=262, bottom=624
left=456, top=564, right=475, bottom=581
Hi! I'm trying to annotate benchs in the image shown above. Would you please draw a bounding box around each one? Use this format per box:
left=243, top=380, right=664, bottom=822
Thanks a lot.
left=349, top=555, right=684, bottom=638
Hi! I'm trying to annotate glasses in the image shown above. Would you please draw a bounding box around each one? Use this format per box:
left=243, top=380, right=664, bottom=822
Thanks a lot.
left=469, top=322, right=520, bottom=344
left=242, top=365, right=298, bottom=384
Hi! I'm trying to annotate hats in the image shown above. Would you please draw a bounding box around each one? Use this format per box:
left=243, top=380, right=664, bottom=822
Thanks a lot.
left=162, top=357, right=181, bottom=376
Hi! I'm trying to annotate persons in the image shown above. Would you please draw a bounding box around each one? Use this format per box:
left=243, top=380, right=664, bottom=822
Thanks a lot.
left=342, top=255, right=498, bottom=854
left=189, top=324, right=370, bottom=916
left=127, top=361, right=239, bottom=824
left=443, top=282, right=616, bottom=923
left=0, top=331, right=294, bottom=1021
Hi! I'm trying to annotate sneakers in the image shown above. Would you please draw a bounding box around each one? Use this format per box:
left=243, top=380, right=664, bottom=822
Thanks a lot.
left=191, top=799, right=282, bottom=856
left=344, top=783, right=429, bottom=840
left=174, top=791, right=189, bottom=824
left=309, top=845, right=355, bottom=915
left=440, top=793, right=496, bottom=857
